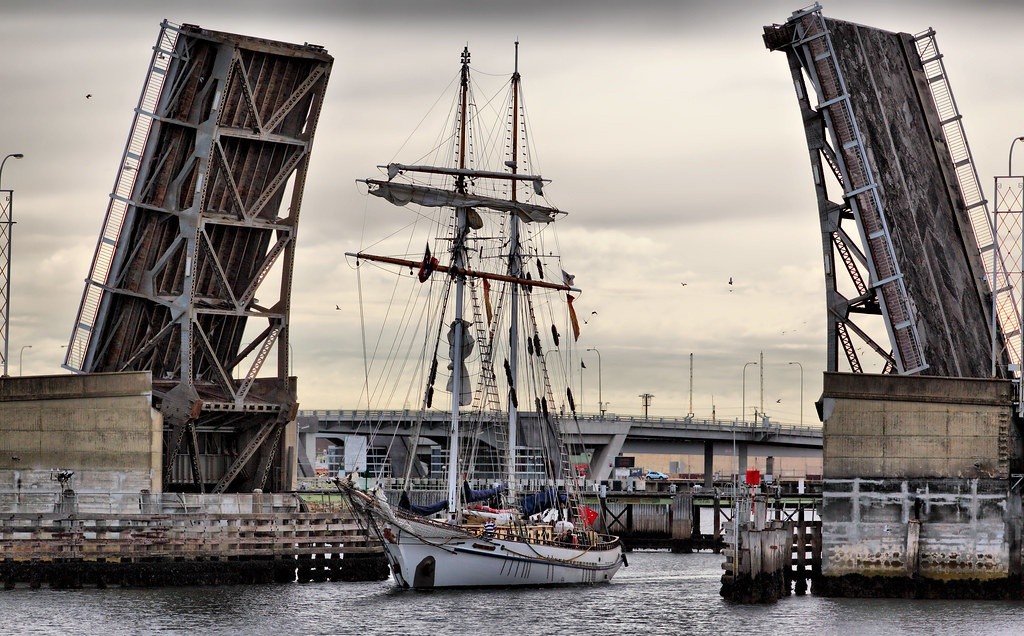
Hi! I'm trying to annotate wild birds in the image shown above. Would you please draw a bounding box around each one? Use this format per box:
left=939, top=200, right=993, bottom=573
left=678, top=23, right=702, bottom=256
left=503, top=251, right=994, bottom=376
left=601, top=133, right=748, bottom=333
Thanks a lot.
left=728, top=277, right=732, bottom=285
left=681, top=282, right=687, bottom=286
left=86, top=94, right=91, bottom=99
left=776, top=399, right=781, bottom=403
left=592, top=312, right=597, bottom=314
left=857, top=348, right=864, bottom=355
left=336, top=305, right=341, bottom=310
left=584, top=320, right=588, bottom=324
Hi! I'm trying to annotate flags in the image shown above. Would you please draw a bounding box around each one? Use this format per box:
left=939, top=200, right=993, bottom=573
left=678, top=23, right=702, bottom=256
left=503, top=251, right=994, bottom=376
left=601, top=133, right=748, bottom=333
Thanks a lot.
left=562, top=270, right=575, bottom=284
left=482, top=278, right=493, bottom=326
left=567, top=293, right=580, bottom=341
left=417, top=243, right=440, bottom=283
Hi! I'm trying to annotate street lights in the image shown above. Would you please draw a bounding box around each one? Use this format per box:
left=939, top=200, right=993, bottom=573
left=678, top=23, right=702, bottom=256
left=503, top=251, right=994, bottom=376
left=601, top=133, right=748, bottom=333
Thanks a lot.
left=587, top=349, right=601, bottom=419
left=0, top=153, right=25, bottom=189
left=742, top=362, right=757, bottom=425
left=789, top=361, right=803, bottom=428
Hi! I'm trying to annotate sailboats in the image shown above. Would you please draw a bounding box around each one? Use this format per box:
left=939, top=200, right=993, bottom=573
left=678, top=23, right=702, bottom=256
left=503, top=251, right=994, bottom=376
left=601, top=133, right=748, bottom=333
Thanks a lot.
left=328, top=38, right=624, bottom=591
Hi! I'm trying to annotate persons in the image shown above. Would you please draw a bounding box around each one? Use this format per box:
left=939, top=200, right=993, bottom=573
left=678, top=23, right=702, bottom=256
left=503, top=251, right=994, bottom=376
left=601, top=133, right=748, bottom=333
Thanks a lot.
left=509, top=512, right=574, bottom=548
left=484, top=518, right=497, bottom=539
left=560, top=502, right=572, bottom=520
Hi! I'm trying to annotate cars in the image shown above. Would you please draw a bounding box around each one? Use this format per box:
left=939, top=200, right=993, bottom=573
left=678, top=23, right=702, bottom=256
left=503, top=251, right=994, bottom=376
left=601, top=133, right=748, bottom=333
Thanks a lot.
left=645, top=472, right=668, bottom=481
